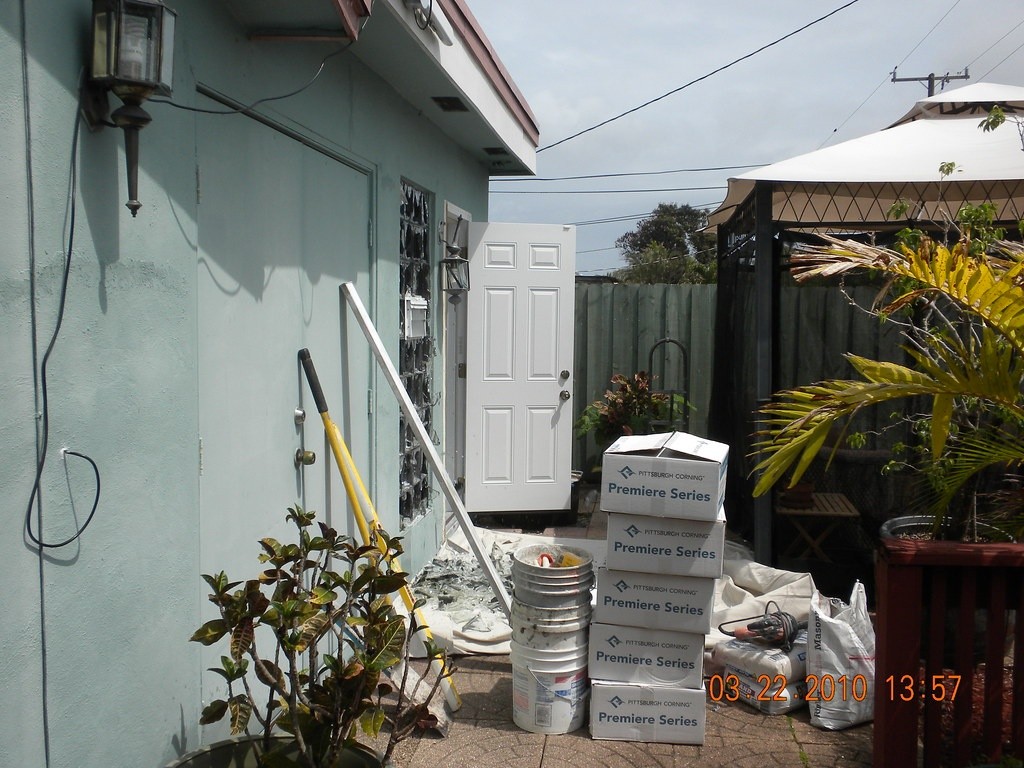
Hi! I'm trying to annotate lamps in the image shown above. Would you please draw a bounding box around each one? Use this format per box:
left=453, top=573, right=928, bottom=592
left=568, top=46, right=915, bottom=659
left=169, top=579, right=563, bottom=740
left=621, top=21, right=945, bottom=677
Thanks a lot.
left=81, top=1, right=182, bottom=218
left=437, top=213, right=469, bottom=312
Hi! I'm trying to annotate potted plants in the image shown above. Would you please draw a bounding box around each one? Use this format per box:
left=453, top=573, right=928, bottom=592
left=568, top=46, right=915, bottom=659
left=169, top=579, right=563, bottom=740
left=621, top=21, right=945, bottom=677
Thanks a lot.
left=162, top=503, right=459, bottom=768
left=780, top=476, right=816, bottom=511
left=744, top=162, right=1023, bottom=671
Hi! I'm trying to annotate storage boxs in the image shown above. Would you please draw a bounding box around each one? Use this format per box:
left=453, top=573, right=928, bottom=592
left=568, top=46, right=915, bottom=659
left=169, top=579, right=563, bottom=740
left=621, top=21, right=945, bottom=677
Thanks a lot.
left=594, top=561, right=717, bottom=634
left=606, top=506, right=727, bottom=579
left=599, top=430, right=730, bottom=523
left=588, top=678, right=709, bottom=746
left=588, top=617, right=706, bottom=691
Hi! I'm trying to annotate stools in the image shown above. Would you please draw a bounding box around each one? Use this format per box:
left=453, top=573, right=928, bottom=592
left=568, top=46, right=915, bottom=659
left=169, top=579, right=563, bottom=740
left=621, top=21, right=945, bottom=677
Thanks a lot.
left=774, top=492, right=862, bottom=564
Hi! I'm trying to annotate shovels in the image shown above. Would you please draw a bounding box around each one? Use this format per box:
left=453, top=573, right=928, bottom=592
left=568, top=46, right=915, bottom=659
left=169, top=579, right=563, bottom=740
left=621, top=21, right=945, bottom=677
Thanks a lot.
left=296, top=347, right=453, bottom=740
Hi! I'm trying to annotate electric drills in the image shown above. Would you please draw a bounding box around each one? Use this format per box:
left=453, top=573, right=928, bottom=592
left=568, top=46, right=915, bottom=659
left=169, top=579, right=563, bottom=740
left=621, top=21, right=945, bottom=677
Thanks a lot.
left=718, top=600, right=808, bottom=653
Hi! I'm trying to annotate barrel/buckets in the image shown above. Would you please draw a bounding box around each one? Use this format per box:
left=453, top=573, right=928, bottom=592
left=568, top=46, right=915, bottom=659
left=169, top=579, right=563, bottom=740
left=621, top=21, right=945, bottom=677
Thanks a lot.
left=510, top=544, right=596, bottom=735
